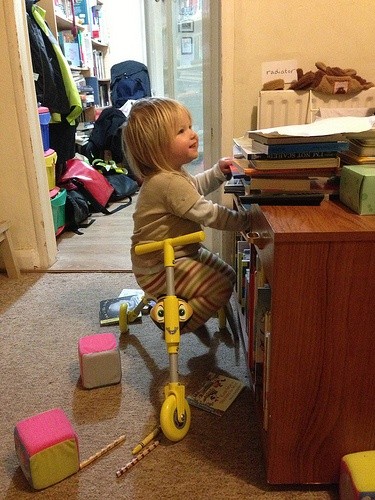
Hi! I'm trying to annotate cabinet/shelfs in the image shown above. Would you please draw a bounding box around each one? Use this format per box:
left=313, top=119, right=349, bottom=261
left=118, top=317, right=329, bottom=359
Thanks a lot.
left=231, top=194, right=375, bottom=485
left=34, top=0, right=112, bottom=124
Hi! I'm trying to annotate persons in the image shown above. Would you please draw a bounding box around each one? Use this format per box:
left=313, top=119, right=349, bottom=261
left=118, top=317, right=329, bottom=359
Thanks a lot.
left=122, top=96, right=253, bottom=340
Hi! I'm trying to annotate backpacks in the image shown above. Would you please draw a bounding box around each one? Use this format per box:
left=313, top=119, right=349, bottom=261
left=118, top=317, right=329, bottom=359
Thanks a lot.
left=66, top=190, right=95, bottom=232
left=85, top=107, right=129, bottom=166
left=109, top=60, right=152, bottom=108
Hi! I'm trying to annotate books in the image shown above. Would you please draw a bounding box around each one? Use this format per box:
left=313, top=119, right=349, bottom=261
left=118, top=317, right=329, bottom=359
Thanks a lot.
left=98, top=287, right=153, bottom=326
left=232, top=233, right=270, bottom=414
left=187, top=372, right=245, bottom=417
left=55, top=1, right=111, bottom=145
left=222, top=114, right=375, bottom=194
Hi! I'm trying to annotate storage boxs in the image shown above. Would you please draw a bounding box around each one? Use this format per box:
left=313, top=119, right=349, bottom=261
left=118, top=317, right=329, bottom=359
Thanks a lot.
left=37, top=107, right=67, bottom=238
left=340, top=165, right=375, bottom=216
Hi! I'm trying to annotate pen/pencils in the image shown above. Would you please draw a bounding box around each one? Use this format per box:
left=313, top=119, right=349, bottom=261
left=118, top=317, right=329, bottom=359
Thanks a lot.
left=132, top=424, right=161, bottom=454
left=116, top=439, right=161, bottom=478
left=79, top=435, right=126, bottom=468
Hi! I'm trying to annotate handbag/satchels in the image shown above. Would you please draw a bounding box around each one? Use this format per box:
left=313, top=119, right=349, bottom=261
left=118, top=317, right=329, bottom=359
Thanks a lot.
left=105, top=172, right=137, bottom=202
left=58, top=158, right=115, bottom=211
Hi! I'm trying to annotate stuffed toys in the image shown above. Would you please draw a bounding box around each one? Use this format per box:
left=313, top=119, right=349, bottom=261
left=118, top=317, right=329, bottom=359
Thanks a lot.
left=288, top=62, right=374, bottom=95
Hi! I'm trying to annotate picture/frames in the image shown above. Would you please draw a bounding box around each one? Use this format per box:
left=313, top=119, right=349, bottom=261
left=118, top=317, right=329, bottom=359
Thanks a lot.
left=177, top=20, right=194, bottom=33
left=181, top=37, right=192, bottom=55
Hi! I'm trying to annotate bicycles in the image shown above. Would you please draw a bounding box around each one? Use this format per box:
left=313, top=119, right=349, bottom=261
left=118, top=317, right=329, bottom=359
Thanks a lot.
left=118, top=231, right=228, bottom=442
